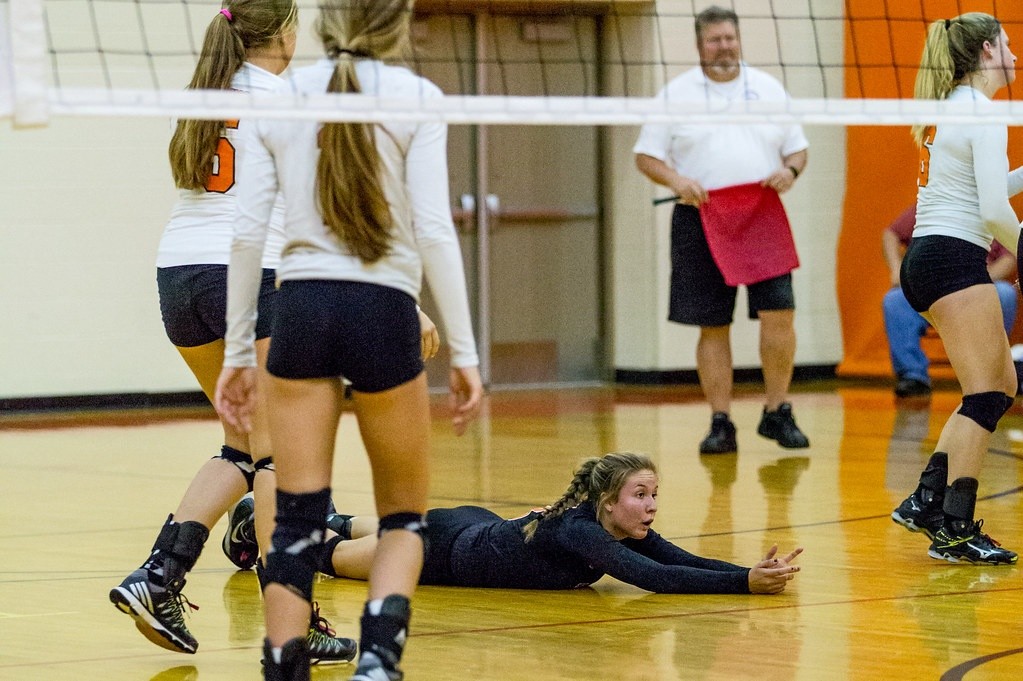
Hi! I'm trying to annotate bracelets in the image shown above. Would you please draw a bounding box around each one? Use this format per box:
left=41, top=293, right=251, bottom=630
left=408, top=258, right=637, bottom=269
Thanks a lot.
left=788, top=165, right=799, bottom=179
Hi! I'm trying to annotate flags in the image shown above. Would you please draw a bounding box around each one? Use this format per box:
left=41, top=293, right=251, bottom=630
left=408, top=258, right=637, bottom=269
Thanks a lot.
left=697, top=177, right=802, bottom=289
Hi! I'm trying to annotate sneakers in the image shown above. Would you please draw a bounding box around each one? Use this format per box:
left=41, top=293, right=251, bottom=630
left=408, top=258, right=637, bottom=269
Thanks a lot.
left=758, top=401, right=811, bottom=449
left=348, top=651, right=404, bottom=681
left=928, top=522, right=1018, bottom=566
left=109, top=569, right=198, bottom=655
left=892, top=494, right=941, bottom=541
left=308, top=627, right=358, bottom=666
left=222, top=495, right=260, bottom=569
left=699, top=412, right=737, bottom=455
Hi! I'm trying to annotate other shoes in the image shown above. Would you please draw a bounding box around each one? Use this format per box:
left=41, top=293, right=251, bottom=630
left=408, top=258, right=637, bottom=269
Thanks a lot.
left=893, top=380, right=932, bottom=399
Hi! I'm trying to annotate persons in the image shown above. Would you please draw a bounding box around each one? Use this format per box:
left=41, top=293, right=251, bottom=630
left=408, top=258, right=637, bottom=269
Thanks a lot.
left=219, top=451, right=804, bottom=597
left=213, top=0, right=486, bottom=681
left=633, top=6, right=815, bottom=455
left=104, top=0, right=358, bottom=667
left=888, top=9, right=1022, bottom=568
left=879, top=202, right=1018, bottom=398
